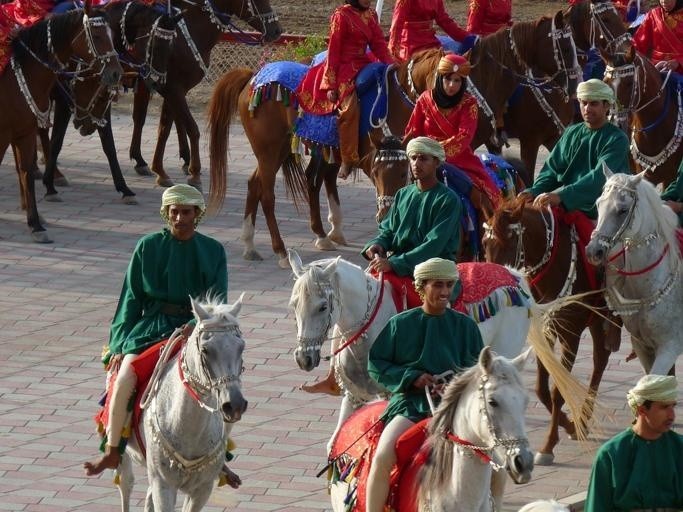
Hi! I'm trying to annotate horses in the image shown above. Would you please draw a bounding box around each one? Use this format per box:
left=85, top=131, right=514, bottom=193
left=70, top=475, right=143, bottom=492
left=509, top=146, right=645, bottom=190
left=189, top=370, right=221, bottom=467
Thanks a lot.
left=285, top=246, right=651, bottom=460
left=328, top=344, right=536, bottom=512
left=483, top=193, right=625, bottom=465
left=1, top=1, right=122, bottom=245
left=102, top=0, right=189, bottom=179
left=104, top=292, right=250, bottom=512
left=208, top=47, right=496, bottom=270
left=151, top=0, right=284, bottom=197
left=513, top=0, right=637, bottom=187
left=596, top=40, right=682, bottom=189
left=42, top=54, right=142, bottom=204
left=586, top=159, right=683, bottom=375
left=298, top=9, right=584, bottom=252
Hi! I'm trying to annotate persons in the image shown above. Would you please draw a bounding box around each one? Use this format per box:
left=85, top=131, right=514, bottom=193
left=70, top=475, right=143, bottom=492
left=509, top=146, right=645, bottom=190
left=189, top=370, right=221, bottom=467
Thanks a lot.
left=633, top=1, right=682, bottom=72
left=569, top=0, right=635, bottom=79
left=366, top=258, right=508, bottom=511
left=300, top=137, right=462, bottom=396
left=467, top=1, right=513, bottom=38
left=517, top=80, right=630, bottom=352
left=319, top=1, right=403, bottom=180
left=585, top=375, right=681, bottom=511
left=47, top=0, right=92, bottom=90
left=83, top=184, right=242, bottom=488
left=626, top=158, right=683, bottom=364
left=406, top=54, right=503, bottom=210
left=388, top=0, right=470, bottom=63
